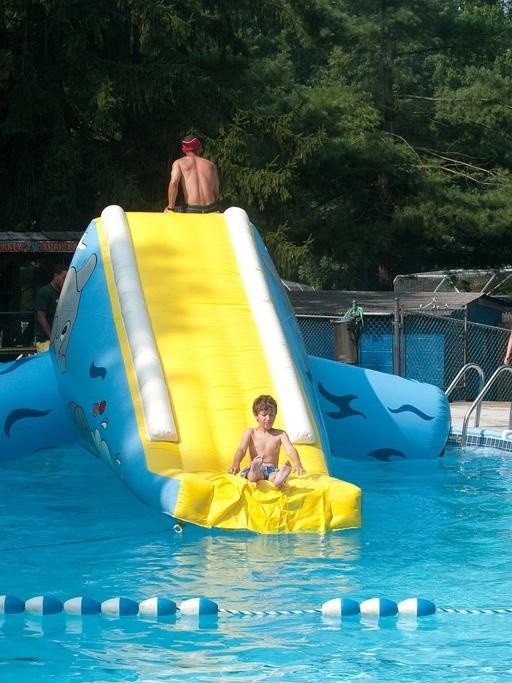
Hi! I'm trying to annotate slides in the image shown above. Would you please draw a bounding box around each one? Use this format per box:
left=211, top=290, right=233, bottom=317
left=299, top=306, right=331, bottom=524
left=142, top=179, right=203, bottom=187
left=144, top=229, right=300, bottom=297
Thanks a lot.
left=96, top=205, right=361, bottom=531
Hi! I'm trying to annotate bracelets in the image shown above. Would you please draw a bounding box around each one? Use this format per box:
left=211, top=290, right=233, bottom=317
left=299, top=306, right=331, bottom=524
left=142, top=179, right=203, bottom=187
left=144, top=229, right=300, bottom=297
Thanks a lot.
left=167, top=206, right=174, bottom=211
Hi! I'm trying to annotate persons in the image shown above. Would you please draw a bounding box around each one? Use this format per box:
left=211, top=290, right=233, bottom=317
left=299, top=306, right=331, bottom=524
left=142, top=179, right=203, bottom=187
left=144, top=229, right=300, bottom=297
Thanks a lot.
left=504, top=334, right=512, bottom=367
left=35, top=267, right=69, bottom=352
left=164, top=135, right=221, bottom=212
left=230, top=394, right=306, bottom=488
